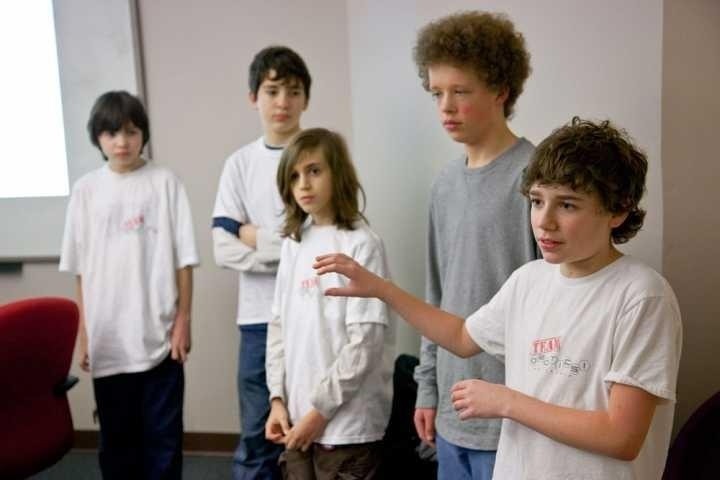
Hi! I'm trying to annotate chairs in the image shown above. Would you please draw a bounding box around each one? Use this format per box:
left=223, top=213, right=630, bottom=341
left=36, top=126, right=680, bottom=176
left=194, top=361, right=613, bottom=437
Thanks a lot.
left=0, top=298, right=85, bottom=479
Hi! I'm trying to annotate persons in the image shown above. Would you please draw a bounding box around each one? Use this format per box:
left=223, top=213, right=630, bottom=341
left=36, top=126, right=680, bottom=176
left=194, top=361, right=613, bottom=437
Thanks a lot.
left=313, top=115, right=683, bottom=480
left=58, top=91, right=200, bottom=480
left=265, top=128, right=397, bottom=480
left=210, top=45, right=311, bottom=480
left=411, top=9, right=543, bottom=480
